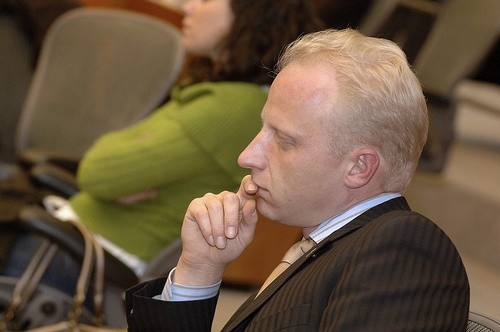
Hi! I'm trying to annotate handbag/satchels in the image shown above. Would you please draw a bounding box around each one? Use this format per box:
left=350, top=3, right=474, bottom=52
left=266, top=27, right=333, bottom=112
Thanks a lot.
left=1, top=222, right=126, bottom=331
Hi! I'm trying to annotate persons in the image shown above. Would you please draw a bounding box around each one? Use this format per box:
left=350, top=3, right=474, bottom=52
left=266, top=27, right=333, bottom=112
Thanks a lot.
left=4, top=0, right=332, bottom=316
left=124, top=27, right=472, bottom=332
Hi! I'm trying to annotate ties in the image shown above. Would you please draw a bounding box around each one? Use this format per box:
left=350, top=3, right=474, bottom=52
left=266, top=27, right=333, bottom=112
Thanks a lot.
left=253, top=237, right=314, bottom=300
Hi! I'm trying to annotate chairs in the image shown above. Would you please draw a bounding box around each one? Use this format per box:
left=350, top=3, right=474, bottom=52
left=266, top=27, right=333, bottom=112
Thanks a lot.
left=0, top=0, right=500, bottom=332
